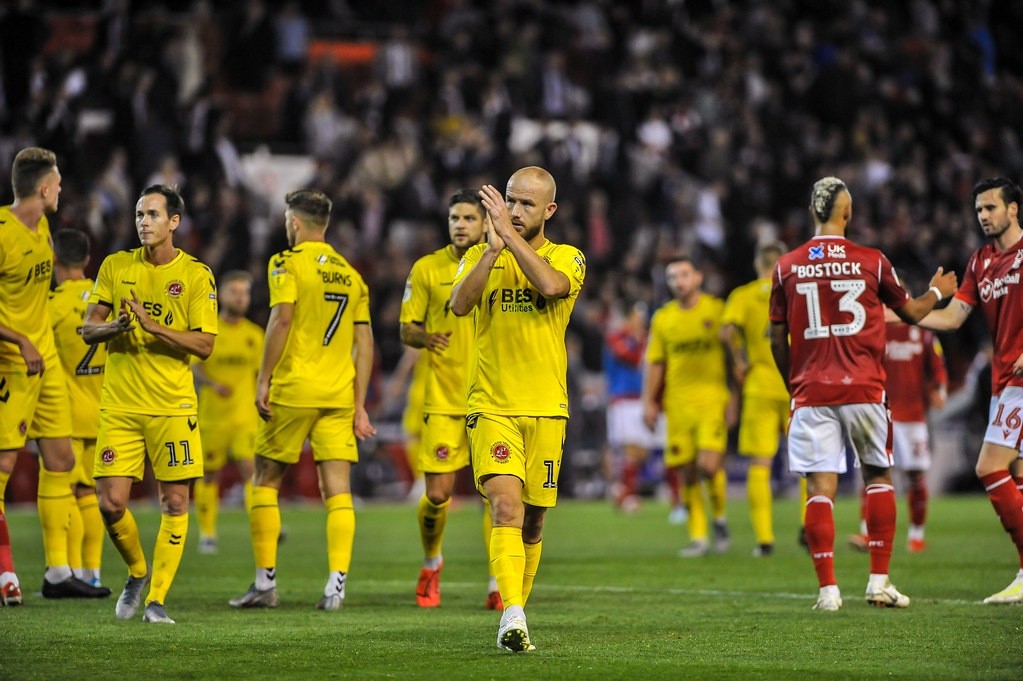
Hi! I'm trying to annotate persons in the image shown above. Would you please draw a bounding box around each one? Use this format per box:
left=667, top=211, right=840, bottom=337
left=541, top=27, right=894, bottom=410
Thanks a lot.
left=188, top=270, right=287, bottom=554
left=845, top=274, right=948, bottom=552
left=912, top=172, right=1023, bottom=606
left=81, top=184, right=219, bottom=624
left=227, top=189, right=378, bottom=611
left=449, top=166, right=587, bottom=651
left=767, top=177, right=958, bottom=611
left=641, top=255, right=744, bottom=561
left=0, top=508, right=23, bottom=607
left=721, top=241, right=813, bottom=560
left=0, top=0, right=1022, bottom=503
left=603, top=305, right=689, bottom=526
left=398, top=190, right=505, bottom=610
left=45, top=227, right=110, bottom=587
left=0, top=145, right=114, bottom=599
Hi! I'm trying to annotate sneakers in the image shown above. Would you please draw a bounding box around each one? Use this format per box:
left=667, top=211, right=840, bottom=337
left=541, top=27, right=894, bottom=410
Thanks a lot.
left=865, top=579, right=910, bottom=609
left=487, top=591, right=504, bottom=610
left=416, top=560, right=444, bottom=608
left=812, top=590, right=842, bottom=611
left=116, top=572, right=151, bottom=619
left=142, top=600, right=176, bottom=624
left=500, top=614, right=535, bottom=651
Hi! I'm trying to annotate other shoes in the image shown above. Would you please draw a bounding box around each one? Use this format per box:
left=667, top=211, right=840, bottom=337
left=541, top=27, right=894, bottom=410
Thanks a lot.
left=848, top=534, right=869, bottom=554
left=908, top=538, right=927, bottom=552
left=42, top=575, right=112, bottom=600
left=315, top=591, right=345, bottom=610
left=983, top=565, right=1023, bottom=602
left=678, top=539, right=707, bottom=558
left=714, top=520, right=733, bottom=552
left=229, top=584, right=278, bottom=608
left=0, top=582, right=23, bottom=606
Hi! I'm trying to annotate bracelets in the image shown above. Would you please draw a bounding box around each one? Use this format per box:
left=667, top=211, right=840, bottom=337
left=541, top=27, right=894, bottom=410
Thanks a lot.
left=928, top=286, right=942, bottom=301
